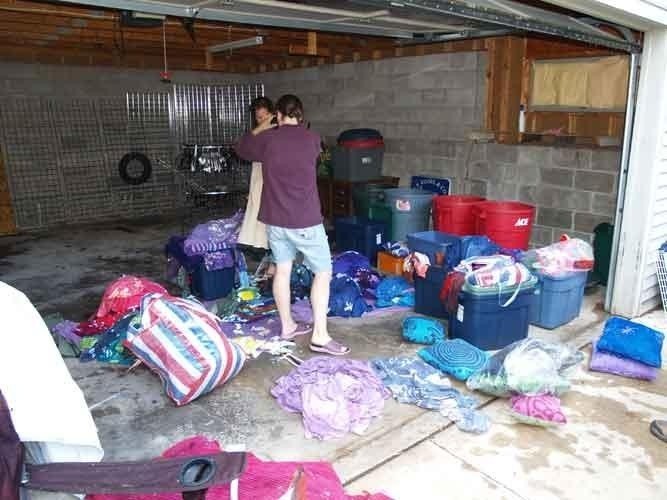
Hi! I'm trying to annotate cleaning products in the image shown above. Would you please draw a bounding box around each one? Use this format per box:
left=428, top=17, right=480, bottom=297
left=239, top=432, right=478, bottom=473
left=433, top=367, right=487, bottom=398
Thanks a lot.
left=518, top=103, right=526, bottom=133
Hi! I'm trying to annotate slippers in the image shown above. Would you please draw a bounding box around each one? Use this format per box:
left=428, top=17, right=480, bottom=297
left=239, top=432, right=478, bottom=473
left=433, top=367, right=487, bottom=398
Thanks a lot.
left=308, top=339, right=351, bottom=356
left=280, top=323, right=312, bottom=339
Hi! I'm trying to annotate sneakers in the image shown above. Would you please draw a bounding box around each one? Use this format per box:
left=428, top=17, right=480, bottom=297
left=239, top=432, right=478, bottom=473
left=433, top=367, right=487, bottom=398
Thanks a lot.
left=650, top=419, right=667, bottom=443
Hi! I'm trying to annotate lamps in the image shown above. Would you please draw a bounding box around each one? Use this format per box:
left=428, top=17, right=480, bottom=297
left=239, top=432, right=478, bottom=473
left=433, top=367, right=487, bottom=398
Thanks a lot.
left=207, top=25, right=263, bottom=53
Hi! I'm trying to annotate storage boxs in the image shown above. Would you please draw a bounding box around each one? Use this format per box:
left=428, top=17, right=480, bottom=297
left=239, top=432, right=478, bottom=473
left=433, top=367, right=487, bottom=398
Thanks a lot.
left=529, top=273, right=588, bottom=328
left=316, top=144, right=535, bottom=315
left=447, top=273, right=535, bottom=351
left=181, top=248, right=235, bottom=301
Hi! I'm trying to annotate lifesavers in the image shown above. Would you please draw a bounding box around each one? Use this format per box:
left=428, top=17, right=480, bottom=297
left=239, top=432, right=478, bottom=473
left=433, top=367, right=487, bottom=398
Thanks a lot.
left=118, top=152, right=151, bottom=185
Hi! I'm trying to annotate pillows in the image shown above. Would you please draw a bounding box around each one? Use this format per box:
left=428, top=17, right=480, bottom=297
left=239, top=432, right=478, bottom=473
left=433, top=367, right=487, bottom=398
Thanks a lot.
left=401, top=313, right=665, bottom=428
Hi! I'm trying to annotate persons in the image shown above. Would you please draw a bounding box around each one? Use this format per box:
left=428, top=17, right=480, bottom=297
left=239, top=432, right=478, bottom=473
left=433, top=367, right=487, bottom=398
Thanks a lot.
left=235, top=96, right=278, bottom=280
left=232, top=94, right=351, bottom=357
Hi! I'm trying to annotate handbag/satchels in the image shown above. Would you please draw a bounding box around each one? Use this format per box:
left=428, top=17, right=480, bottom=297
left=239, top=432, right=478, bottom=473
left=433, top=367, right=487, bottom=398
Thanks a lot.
left=123, top=293, right=246, bottom=407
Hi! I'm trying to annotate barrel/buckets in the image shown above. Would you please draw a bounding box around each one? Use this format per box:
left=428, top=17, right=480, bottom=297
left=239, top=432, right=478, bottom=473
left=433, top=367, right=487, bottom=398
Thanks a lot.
left=383, top=187, right=432, bottom=241
left=476, top=200, right=535, bottom=251
left=434, top=194, right=486, bottom=236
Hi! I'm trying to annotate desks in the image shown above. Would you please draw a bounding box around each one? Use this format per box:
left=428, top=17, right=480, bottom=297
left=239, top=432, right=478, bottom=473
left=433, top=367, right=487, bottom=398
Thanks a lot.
left=185, top=186, right=248, bottom=219
